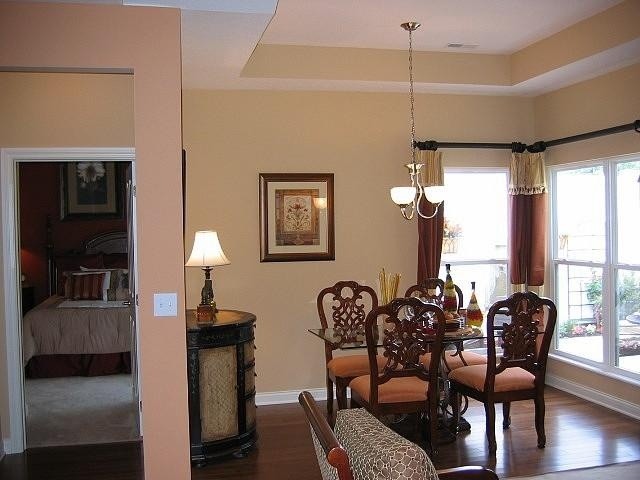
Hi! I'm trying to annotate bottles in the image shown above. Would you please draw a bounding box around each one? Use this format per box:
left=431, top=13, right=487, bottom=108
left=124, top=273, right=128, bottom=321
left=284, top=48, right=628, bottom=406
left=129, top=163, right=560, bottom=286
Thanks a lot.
left=465, top=281, right=484, bottom=327
left=444, top=264, right=457, bottom=313
left=196, top=291, right=216, bottom=324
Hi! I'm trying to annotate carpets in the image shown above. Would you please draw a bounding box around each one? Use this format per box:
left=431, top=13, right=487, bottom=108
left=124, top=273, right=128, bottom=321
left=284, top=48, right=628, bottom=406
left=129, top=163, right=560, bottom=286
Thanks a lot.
left=23, top=374, right=142, bottom=450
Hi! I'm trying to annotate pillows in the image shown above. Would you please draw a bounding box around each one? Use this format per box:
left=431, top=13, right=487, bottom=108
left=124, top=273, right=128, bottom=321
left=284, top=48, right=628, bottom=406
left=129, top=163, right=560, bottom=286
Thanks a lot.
left=56, top=249, right=130, bottom=303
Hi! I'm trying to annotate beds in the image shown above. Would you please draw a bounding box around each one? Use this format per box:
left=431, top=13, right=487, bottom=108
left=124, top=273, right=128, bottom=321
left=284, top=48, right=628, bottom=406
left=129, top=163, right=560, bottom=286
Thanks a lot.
left=23, top=229, right=132, bottom=380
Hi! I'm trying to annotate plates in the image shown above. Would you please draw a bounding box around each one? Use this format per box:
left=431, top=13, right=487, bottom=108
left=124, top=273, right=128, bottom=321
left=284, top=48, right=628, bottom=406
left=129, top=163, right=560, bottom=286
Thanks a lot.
left=432, top=319, right=460, bottom=333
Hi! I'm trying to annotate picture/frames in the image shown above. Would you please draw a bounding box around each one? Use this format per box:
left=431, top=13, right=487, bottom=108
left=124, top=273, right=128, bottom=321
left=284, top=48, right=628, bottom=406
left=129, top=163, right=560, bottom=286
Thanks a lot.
left=258, top=171, right=336, bottom=263
left=58, top=161, right=124, bottom=223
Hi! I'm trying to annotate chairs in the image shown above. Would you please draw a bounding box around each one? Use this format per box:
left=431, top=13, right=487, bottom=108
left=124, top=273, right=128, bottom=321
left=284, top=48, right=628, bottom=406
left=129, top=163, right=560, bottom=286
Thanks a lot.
left=448, top=291, right=557, bottom=455
left=316, top=278, right=462, bottom=460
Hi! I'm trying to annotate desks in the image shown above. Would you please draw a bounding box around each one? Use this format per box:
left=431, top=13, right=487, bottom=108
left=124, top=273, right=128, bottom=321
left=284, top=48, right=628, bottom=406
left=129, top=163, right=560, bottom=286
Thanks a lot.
left=308, top=312, right=547, bottom=445
left=185, top=308, right=259, bottom=471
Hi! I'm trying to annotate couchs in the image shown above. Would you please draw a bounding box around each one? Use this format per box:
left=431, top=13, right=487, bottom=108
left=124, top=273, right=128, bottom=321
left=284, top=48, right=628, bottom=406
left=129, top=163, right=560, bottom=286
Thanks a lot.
left=298, top=391, right=499, bottom=480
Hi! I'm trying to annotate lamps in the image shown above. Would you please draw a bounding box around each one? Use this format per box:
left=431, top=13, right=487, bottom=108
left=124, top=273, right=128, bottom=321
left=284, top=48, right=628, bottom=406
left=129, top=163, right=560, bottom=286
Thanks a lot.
left=389, top=21, right=450, bottom=222
left=183, top=229, right=233, bottom=313
